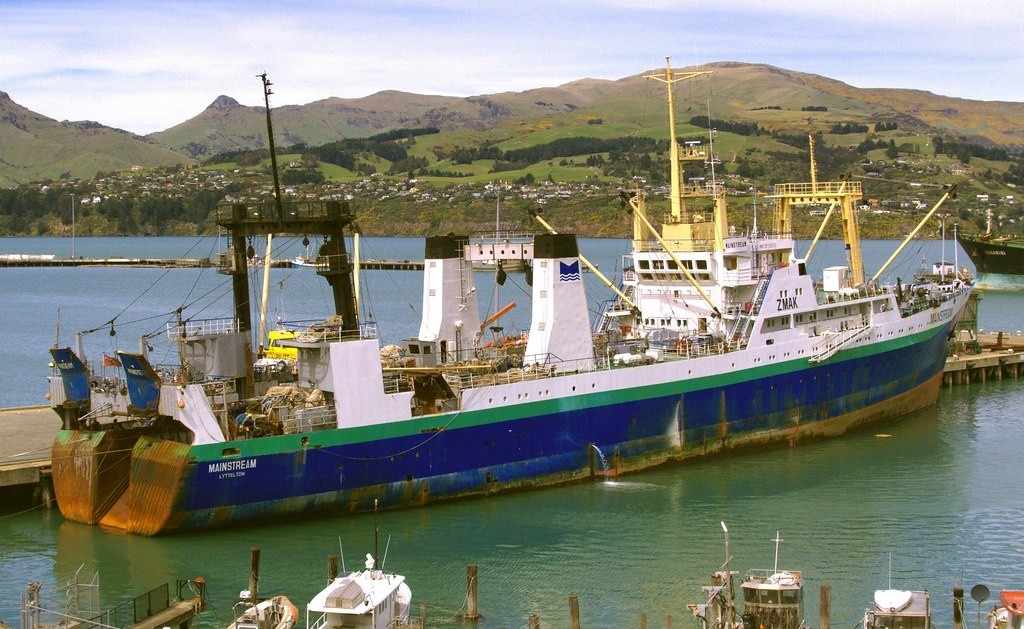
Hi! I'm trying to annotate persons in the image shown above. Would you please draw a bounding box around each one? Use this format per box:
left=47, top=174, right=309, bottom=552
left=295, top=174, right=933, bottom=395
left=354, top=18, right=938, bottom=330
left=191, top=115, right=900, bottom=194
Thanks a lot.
left=728, top=223, right=737, bottom=237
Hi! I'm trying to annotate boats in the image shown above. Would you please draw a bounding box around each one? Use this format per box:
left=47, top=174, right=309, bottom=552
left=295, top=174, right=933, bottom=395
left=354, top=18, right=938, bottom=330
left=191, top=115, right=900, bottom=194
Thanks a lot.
left=305, top=499, right=426, bottom=629
left=226, top=593, right=297, bottom=629
left=956, top=205, right=1024, bottom=274
left=863, top=589, right=931, bottom=629
left=988, top=586, right=1024, bottom=629
left=684, top=521, right=808, bottom=629
left=49, top=57, right=976, bottom=535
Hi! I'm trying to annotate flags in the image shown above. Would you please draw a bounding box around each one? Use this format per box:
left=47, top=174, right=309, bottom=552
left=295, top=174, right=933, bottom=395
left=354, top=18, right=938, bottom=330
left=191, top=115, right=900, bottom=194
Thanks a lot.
left=103, top=353, right=122, bottom=367
